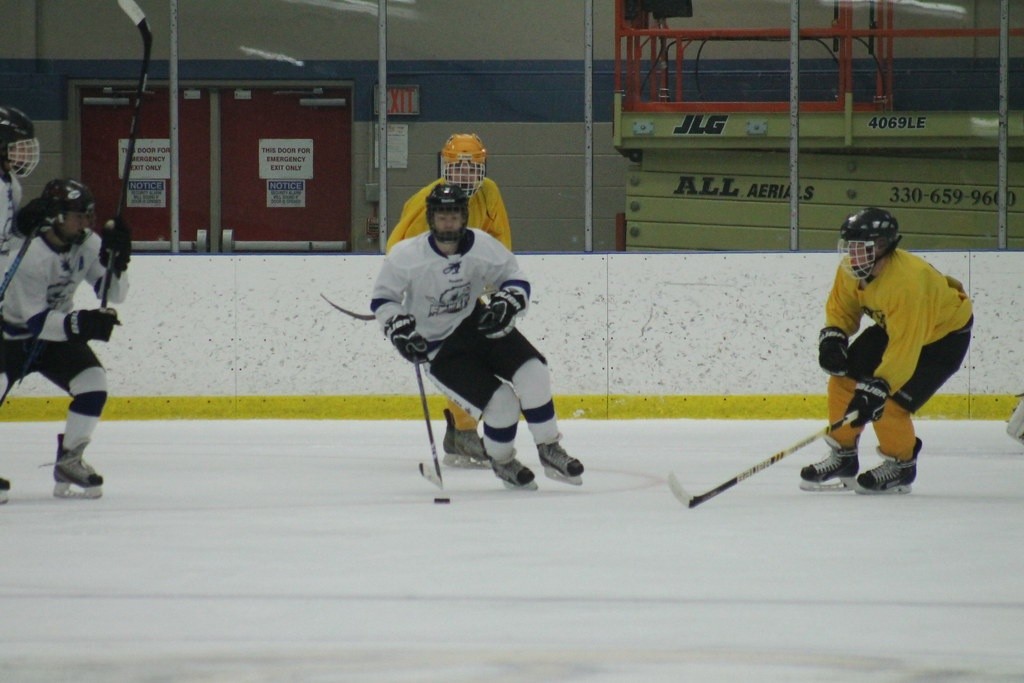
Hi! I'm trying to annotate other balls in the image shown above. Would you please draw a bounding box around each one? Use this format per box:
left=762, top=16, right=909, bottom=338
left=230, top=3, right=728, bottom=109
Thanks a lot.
left=433, top=498, right=450, bottom=503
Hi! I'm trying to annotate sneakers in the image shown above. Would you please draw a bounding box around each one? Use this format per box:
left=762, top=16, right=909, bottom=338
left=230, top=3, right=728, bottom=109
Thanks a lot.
left=856, top=437, right=922, bottom=495
left=0, top=479, right=10, bottom=504
left=479, top=437, right=538, bottom=490
left=536, top=431, right=585, bottom=485
left=443, top=409, right=491, bottom=468
left=54, top=433, right=104, bottom=498
left=799, top=434, right=862, bottom=492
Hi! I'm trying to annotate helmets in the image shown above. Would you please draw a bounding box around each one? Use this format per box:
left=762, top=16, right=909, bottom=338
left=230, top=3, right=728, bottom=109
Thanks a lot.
left=441, top=134, right=487, bottom=198
left=840, top=208, right=903, bottom=282
left=41, top=178, right=95, bottom=246
left=0, top=106, right=41, bottom=177
left=425, top=183, right=468, bottom=242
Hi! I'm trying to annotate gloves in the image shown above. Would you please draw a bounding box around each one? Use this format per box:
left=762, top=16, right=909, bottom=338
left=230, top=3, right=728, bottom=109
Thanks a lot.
left=818, top=326, right=849, bottom=376
left=383, top=313, right=429, bottom=364
left=98, top=215, right=132, bottom=280
left=11, top=198, right=48, bottom=239
left=476, top=288, right=526, bottom=339
left=63, top=308, right=121, bottom=344
left=843, top=377, right=891, bottom=428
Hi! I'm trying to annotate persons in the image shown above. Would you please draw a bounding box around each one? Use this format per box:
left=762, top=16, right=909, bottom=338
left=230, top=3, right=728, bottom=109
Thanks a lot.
left=799, top=206, right=974, bottom=496
left=0, top=179, right=131, bottom=504
left=371, top=183, right=585, bottom=492
left=387, top=133, right=511, bottom=469
left=0, top=109, right=41, bottom=244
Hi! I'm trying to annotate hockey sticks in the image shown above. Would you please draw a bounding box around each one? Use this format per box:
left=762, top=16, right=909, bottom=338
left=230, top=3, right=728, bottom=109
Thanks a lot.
left=409, top=346, right=444, bottom=489
left=1, top=221, right=42, bottom=298
left=665, top=409, right=858, bottom=510
left=100, top=1, right=154, bottom=313
